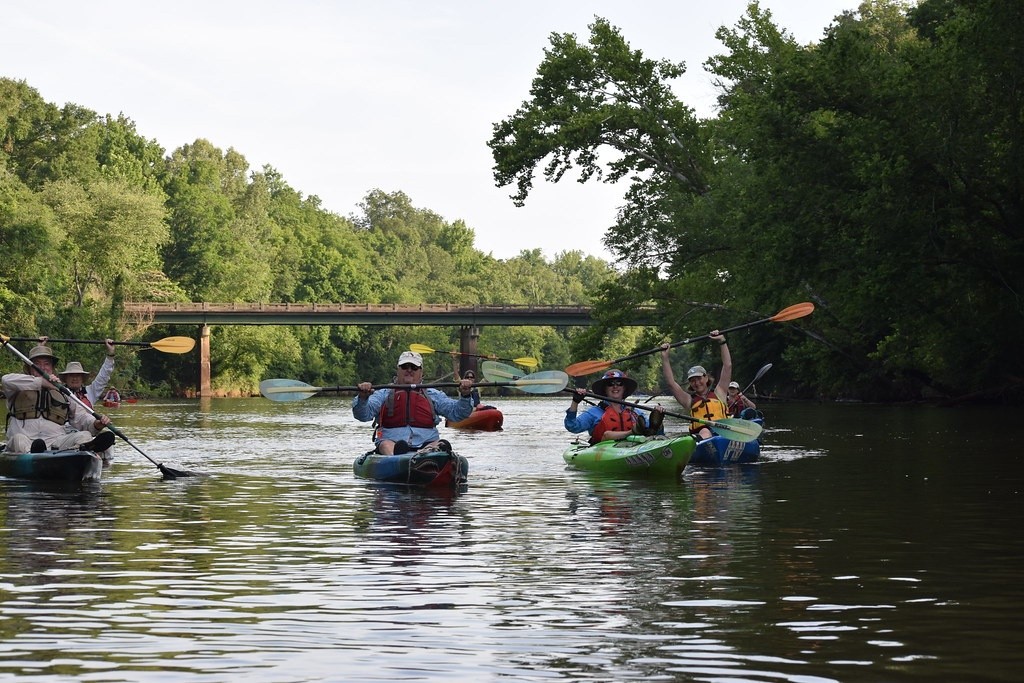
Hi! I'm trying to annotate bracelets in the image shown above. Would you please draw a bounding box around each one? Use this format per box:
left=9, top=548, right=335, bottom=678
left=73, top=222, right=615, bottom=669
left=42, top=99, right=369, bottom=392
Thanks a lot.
left=107, top=354, right=115, bottom=357
left=719, top=339, right=727, bottom=345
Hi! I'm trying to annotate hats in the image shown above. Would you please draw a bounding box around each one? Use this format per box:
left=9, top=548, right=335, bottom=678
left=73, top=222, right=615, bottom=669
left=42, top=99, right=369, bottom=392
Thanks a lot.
left=687, top=366, right=706, bottom=380
left=397, top=351, right=424, bottom=369
left=22, top=346, right=60, bottom=375
left=109, top=386, right=116, bottom=389
left=591, top=369, right=637, bottom=399
left=728, top=382, right=740, bottom=392
left=57, top=361, right=90, bottom=384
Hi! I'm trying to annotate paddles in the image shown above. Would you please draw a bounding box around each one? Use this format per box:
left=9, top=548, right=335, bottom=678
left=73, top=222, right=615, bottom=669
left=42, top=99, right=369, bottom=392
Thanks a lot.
left=258, top=371, right=569, bottom=404
left=0, top=335, right=196, bottom=355
left=98, top=399, right=137, bottom=403
left=565, top=302, right=816, bottom=377
left=409, top=342, right=539, bottom=368
left=0, top=338, right=193, bottom=480
left=729, top=364, right=773, bottom=409
left=480, top=359, right=763, bottom=443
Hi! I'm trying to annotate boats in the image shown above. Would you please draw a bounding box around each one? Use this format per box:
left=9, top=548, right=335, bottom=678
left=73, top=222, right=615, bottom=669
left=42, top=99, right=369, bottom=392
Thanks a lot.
left=102, top=401, right=119, bottom=408
left=445, top=403, right=504, bottom=431
left=563, top=434, right=699, bottom=478
left=738, top=407, right=762, bottom=427
left=688, top=435, right=761, bottom=468
left=1, top=443, right=103, bottom=483
left=354, top=450, right=468, bottom=485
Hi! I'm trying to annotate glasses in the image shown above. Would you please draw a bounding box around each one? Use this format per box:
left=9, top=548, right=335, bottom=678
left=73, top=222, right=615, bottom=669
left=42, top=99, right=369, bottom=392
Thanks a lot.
left=398, top=363, right=421, bottom=371
left=603, top=380, right=624, bottom=387
left=464, top=377, right=474, bottom=379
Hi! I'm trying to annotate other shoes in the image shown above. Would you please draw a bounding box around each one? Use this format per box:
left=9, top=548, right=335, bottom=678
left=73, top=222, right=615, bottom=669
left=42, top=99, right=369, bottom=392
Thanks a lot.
left=79, top=431, right=116, bottom=453
left=30, top=439, right=47, bottom=454
left=632, top=414, right=647, bottom=436
left=689, top=434, right=702, bottom=443
left=437, top=439, right=451, bottom=453
left=647, top=410, right=662, bottom=437
left=393, top=440, right=409, bottom=455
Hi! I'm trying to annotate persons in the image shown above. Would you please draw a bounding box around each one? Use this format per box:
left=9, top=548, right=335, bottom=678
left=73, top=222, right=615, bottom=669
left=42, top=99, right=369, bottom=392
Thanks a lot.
left=726, top=382, right=756, bottom=418
left=2, top=346, right=110, bottom=452
left=352, top=352, right=473, bottom=456
left=36, top=336, right=116, bottom=412
left=452, top=354, right=499, bottom=408
left=661, top=330, right=732, bottom=440
left=564, top=368, right=665, bottom=446
left=103, top=386, right=121, bottom=402
